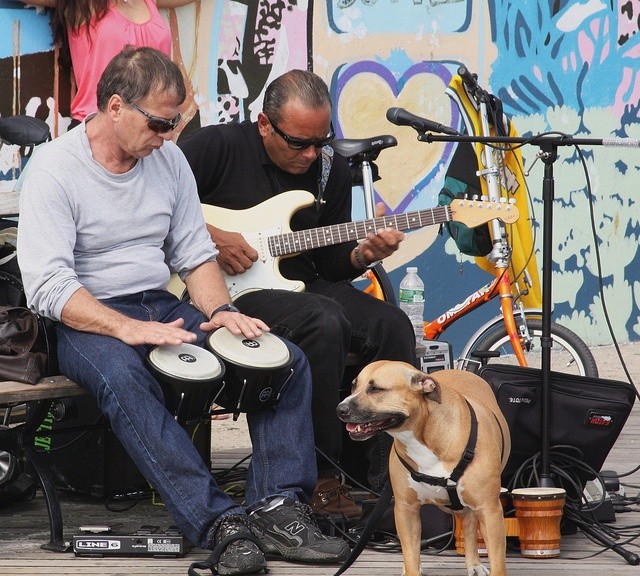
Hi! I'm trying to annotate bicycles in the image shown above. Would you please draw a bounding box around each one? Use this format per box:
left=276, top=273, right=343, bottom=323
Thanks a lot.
left=331, top=66, right=599, bottom=379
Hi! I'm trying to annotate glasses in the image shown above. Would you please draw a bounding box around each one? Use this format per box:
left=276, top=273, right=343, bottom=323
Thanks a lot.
left=129, top=100, right=183, bottom=131
left=266, top=114, right=337, bottom=150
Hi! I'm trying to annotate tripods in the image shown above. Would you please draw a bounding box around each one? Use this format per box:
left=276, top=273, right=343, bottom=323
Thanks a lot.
left=417, top=135, right=640, bottom=566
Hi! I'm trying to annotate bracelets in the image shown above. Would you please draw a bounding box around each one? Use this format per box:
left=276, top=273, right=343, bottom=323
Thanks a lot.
left=355, top=244, right=381, bottom=270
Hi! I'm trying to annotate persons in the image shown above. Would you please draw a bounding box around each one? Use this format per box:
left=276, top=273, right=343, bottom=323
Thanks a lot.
left=15, top=43, right=352, bottom=576
left=17, top=0, right=198, bottom=132
left=173, top=67, right=418, bottom=519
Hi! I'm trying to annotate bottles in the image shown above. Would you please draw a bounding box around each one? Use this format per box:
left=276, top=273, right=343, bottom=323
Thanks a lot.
left=397, top=264, right=425, bottom=349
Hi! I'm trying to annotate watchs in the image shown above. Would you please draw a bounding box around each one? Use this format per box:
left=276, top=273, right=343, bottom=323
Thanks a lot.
left=210, top=304, right=241, bottom=318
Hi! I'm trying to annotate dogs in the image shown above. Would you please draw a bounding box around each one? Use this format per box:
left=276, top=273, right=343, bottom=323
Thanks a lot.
left=336, top=359, right=512, bottom=575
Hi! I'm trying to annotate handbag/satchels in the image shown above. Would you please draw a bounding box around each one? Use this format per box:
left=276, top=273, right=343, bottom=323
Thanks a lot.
left=471, top=365, right=637, bottom=516
left=0, top=242, right=57, bottom=386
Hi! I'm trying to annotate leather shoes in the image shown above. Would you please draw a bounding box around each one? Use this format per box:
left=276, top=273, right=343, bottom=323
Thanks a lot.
left=310, top=475, right=364, bottom=518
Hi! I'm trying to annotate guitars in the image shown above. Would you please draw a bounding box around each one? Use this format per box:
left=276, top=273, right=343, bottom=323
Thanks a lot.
left=162, top=187, right=521, bottom=306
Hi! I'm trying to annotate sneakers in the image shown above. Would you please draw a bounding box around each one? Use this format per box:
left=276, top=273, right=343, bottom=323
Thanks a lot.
left=208, top=514, right=270, bottom=575
left=247, top=496, right=352, bottom=564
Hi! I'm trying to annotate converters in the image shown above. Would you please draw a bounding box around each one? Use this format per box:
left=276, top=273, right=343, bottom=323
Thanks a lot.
left=600, top=470, right=620, bottom=491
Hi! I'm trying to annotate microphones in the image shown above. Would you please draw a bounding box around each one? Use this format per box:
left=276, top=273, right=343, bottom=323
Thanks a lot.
left=386, top=106, right=457, bottom=133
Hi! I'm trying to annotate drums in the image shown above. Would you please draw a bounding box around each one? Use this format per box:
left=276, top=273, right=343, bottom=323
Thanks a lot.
left=511, top=487, right=566, bottom=558
left=144, top=343, right=226, bottom=427
left=204, top=326, right=294, bottom=420
left=455, top=487, right=508, bottom=557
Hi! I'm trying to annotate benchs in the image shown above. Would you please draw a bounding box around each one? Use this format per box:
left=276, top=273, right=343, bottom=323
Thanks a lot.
left=0, top=190, right=98, bottom=551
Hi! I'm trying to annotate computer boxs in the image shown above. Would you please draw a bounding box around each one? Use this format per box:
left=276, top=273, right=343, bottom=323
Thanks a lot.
left=419, top=339, right=454, bottom=374
